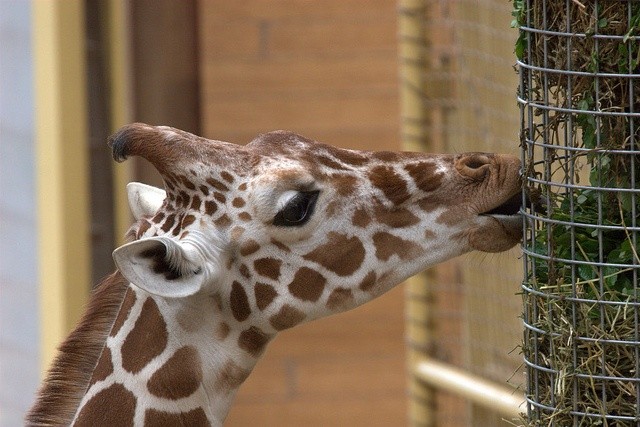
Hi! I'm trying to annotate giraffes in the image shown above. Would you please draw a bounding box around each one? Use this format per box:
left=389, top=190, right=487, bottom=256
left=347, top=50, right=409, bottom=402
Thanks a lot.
left=25, top=122, right=530, bottom=426
